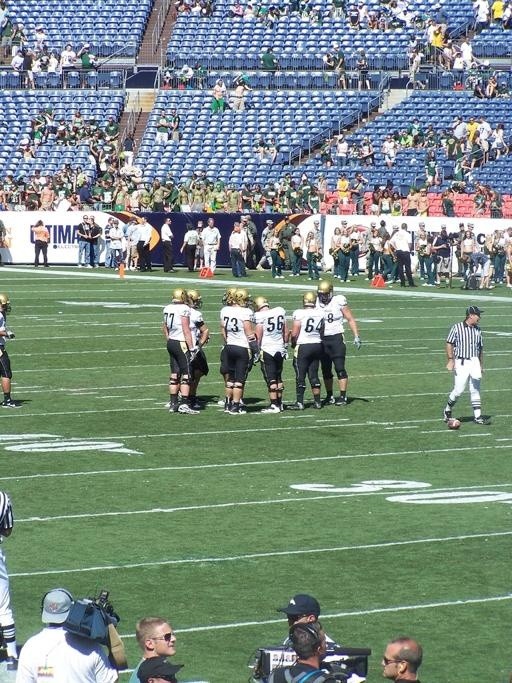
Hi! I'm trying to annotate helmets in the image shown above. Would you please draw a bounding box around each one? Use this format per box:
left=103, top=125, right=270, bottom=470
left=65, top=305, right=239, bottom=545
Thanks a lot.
left=0, top=294, right=12, bottom=314
left=174, top=282, right=333, bottom=306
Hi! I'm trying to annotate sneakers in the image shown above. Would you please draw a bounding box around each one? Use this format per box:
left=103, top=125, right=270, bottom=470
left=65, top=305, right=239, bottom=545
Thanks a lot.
left=167, top=398, right=349, bottom=412
left=442, top=409, right=451, bottom=422
left=473, top=416, right=489, bottom=425
left=6, top=655, right=18, bottom=670
left=1, top=399, right=23, bottom=408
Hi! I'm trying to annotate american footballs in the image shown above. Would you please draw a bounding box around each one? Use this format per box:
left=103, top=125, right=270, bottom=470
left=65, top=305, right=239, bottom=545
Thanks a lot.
left=448, top=420, right=460, bottom=429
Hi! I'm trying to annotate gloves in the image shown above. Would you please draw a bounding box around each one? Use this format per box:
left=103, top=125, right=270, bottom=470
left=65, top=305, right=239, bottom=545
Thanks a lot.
left=353, top=336, right=361, bottom=350
left=189, top=348, right=196, bottom=361
left=6, top=331, right=15, bottom=340
left=252, top=350, right=261, bottom=363
left=195, top=345, right=200, bottom=356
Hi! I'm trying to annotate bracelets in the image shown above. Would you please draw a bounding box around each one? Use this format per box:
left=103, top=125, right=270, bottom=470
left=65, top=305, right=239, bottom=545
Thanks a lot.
left=448, top=356, right=453, bottom=360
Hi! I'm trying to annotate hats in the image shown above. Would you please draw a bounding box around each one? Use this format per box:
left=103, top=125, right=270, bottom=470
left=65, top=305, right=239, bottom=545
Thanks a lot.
left=278, top=595, right=320, bottom=615
left=137, top=656, right=184, bottom=677
left=41, top=588, right=73, bottom=624
left=466, top=305, right=484, bottom=315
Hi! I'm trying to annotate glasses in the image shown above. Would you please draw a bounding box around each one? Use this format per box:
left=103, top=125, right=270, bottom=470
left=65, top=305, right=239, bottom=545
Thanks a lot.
left=384, top=656, right=402, bottom=665
left=150, top=633, right=172, bottom=641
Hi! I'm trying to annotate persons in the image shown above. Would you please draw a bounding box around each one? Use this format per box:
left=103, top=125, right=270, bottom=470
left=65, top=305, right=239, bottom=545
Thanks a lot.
left=277, top=593, right=341, bottom=651
left=380, top=635, right=425, bottom=682
left=443, top=304, right=492, bottom=425
left=17, top=587, right=119, bottom=682
left=1, top=487, right=20, bottom=670
left=1, top=2, right=512, bottom=296
left=161, top=280, right=363, bottom=417
left=129, top=614, right=177, bottom=682
left=260, top=619, right=341, bottom=682
left=1, top=292, right=23, bottom=408
left=135, top=655, right=185, bottom=682
